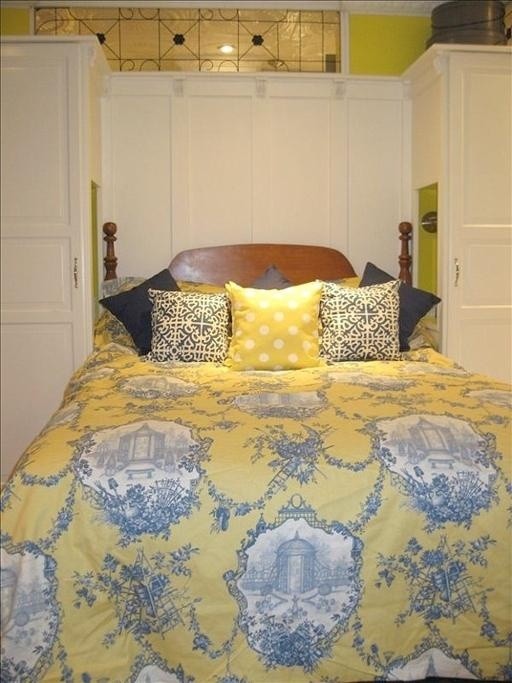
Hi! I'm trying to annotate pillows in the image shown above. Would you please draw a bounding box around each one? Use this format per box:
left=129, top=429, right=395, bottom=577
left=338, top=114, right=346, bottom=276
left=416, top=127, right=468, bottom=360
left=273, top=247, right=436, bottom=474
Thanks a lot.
left=358, top=265, right=440, bottom=351
left=401, top=324, right=438, bottom=362
left=218, top=281, right=323, bottom=371
left=147, top=286, right=231, bottom=362
left=95, top=276, right=227, bottom=349
left=329, top=277, right=362, bottom=288
left=99, top=268, right=182, bottom=355
left=313, top=278, right=404, bottom=361
left=248, top=265, right=293, bottom=289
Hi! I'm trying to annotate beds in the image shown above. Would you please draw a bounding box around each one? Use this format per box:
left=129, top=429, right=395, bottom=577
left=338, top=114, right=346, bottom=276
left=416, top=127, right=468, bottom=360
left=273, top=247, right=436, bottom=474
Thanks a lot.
left=0, top=223, right=512, bottom=681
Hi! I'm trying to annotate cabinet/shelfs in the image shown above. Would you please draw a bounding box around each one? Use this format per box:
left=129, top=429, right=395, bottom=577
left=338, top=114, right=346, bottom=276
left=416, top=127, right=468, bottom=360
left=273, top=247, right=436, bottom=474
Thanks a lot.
left=400, top=44, right=512, bottom=383
left=1, top=35, right=111, bottom=493
